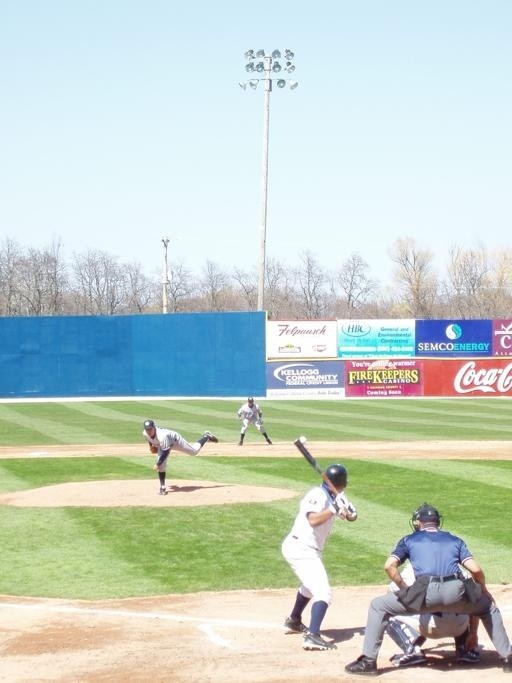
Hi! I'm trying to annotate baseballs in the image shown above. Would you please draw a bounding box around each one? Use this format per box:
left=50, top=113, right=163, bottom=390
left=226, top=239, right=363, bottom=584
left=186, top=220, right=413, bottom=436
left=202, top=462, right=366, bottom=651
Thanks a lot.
left=298, top=436, right=307, bottom=444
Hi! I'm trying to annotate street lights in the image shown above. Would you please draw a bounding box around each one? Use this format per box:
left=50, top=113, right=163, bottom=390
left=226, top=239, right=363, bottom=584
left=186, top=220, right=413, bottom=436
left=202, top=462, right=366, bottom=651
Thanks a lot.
left=237, top=43, right=305, bottom=315
left=158, top=238, right=173, bottom=311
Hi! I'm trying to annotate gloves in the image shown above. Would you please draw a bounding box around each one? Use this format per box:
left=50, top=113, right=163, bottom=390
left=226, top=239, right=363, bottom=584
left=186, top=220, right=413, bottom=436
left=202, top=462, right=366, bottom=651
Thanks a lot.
left=330, top=491, right=348, bottom=513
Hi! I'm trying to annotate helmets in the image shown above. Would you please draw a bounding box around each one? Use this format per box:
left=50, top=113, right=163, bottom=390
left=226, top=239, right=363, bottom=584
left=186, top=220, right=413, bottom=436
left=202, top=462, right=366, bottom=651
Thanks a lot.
left=409, top=503, right=444, bottom=532
left=248, top=397, right=253, bottom=400
left=144, top=419, right=154, bottom=429
left=325, top=464, right=347, bottom=486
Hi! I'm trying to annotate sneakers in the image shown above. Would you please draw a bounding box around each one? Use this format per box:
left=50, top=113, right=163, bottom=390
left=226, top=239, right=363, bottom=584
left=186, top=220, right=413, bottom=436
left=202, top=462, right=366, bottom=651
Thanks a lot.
left=160, top=487, right=167, bottom=495
left=500, top=654, right=512, bottom=671
left=283, top=616, right=310, bottom=633
left=455, top=647, right=481, bottom=662
left=391, top=652, right=427, bottom=667
left=344, top=654, right=377, bottom=675
left=302, top=633, right=336, bottom=650
left=203, top=430, right=218, bottom=443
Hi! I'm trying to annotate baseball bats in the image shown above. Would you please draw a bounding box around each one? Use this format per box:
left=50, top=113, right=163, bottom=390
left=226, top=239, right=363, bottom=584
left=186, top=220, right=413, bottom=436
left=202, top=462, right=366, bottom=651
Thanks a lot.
left=295, top=439, right=340, bottom=499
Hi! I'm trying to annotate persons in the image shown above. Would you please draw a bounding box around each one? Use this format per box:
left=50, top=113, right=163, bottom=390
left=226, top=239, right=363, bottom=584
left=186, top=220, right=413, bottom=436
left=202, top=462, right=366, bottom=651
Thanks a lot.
left=143, top=419, right=218, bottom=495
left=237, top=395, right=272, bottom=446
left=382, top=563, right=481, bottom=668
left=281, top=465, right=356, bottom=650
left=344, top=504, right=511, bottom=677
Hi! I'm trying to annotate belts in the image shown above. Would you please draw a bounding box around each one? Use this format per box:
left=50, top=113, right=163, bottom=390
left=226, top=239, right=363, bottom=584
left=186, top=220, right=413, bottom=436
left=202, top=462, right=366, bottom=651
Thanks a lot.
left=431, top=575, right=454, bottom=582
left=432, top=611, right=442, bottom=616
